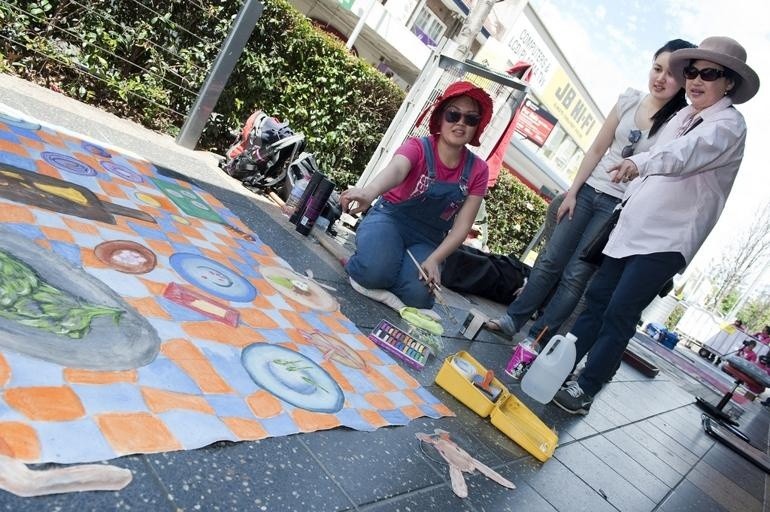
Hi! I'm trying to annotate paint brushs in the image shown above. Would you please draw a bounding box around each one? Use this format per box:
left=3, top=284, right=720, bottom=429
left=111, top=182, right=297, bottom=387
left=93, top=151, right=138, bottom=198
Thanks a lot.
left=406, top=249, right=446, bottom=306
left=419, top=265, right=442, bottom=292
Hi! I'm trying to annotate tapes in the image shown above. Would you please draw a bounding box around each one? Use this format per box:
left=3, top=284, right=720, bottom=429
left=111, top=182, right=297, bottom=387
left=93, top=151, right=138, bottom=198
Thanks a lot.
left=450, top=357, right=476, bottom=380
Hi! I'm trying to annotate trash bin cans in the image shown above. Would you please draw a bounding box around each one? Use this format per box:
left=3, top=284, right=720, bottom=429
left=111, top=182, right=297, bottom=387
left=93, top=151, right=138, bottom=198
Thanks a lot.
left=646, top=321, right=680, bottom=350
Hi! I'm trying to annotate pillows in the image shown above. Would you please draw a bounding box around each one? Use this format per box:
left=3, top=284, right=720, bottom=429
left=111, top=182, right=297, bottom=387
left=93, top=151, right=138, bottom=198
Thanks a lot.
left=725, top=354, right=770, bottom=387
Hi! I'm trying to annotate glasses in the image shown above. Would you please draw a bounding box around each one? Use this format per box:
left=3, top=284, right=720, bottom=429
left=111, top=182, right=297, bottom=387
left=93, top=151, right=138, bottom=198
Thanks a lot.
left=683, top=66, right=728, bottom=80
left=445, top=110, right=479, bottom=127
left=622, top=130, right=641, bottom=158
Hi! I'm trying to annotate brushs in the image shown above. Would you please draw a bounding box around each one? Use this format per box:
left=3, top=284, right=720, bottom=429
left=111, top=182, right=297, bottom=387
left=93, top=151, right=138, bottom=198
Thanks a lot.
left=473, top=369, right=494, bottom=398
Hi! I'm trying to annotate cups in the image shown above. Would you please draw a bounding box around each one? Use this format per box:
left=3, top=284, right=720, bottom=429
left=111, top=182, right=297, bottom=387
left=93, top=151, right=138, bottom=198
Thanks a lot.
left=506, top=338, right=537, bottom=379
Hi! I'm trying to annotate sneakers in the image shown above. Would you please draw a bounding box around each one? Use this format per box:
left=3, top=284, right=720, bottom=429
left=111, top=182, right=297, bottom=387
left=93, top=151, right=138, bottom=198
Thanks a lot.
left=553, top=385, right=594, bottom=416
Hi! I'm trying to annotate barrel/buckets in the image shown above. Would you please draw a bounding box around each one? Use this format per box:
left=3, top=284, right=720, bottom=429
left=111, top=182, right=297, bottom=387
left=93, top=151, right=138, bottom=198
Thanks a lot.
left=644, top=323, right=659, bottom=338
left=658, top=331, right=680, bottom=350
left=520, top=333, right=578, bottom=404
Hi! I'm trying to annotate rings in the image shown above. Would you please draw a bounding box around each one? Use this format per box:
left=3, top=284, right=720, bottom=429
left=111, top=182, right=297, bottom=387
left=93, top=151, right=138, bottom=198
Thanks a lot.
left=625, top=174, right=631, bottom=181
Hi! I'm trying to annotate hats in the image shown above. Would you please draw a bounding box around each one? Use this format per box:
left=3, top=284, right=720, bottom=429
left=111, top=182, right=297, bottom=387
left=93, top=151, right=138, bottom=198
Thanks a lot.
left=429, top=81, right=493, bottom=147
left=668, top=36, right=759, bottom=103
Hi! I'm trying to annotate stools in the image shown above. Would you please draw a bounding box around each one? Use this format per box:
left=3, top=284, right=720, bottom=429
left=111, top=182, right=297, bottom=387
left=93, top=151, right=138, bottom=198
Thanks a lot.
left=693, top=365, right=764, bottom=426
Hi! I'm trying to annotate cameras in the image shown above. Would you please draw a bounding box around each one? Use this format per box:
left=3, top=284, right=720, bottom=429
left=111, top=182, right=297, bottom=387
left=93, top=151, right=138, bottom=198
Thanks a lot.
left=743, top=340, right=750, bottom=346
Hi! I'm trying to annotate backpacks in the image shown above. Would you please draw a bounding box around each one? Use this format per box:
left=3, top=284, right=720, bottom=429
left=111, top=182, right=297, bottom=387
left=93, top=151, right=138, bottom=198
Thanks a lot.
left=218, top=110, right=342, bottom=226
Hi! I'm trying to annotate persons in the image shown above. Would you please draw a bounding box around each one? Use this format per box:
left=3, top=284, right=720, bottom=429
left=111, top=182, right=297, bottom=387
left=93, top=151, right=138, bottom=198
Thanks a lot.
left=479, top=39, right=700, bottom=356
left=551, top=37, right=759, bottom=417
left=754, top=325, right=769, bottom=347
left=719, top=341, right=756, bottom=374
left=707, top=319, right=745, bottom=364
left=734, top=354, right=770, bottom=394
left=337, top=79, right=493, bottom=310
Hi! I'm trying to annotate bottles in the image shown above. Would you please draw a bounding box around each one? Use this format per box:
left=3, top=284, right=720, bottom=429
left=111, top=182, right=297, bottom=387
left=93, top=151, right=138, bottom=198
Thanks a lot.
left=296, top=176, right=335, bottom=237
left=281, top=175, right=310, bottom=218
left=288, top=170, right=326, bottom=225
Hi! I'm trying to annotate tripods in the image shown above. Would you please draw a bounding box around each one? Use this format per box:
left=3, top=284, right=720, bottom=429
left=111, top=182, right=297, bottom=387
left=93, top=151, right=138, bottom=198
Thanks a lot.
left=720, top=346, right=745, bottom=359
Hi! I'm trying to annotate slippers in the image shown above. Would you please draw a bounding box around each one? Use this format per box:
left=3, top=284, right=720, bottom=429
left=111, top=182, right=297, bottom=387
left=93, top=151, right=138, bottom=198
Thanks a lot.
left=484, top=319, right=513, bottom=341
